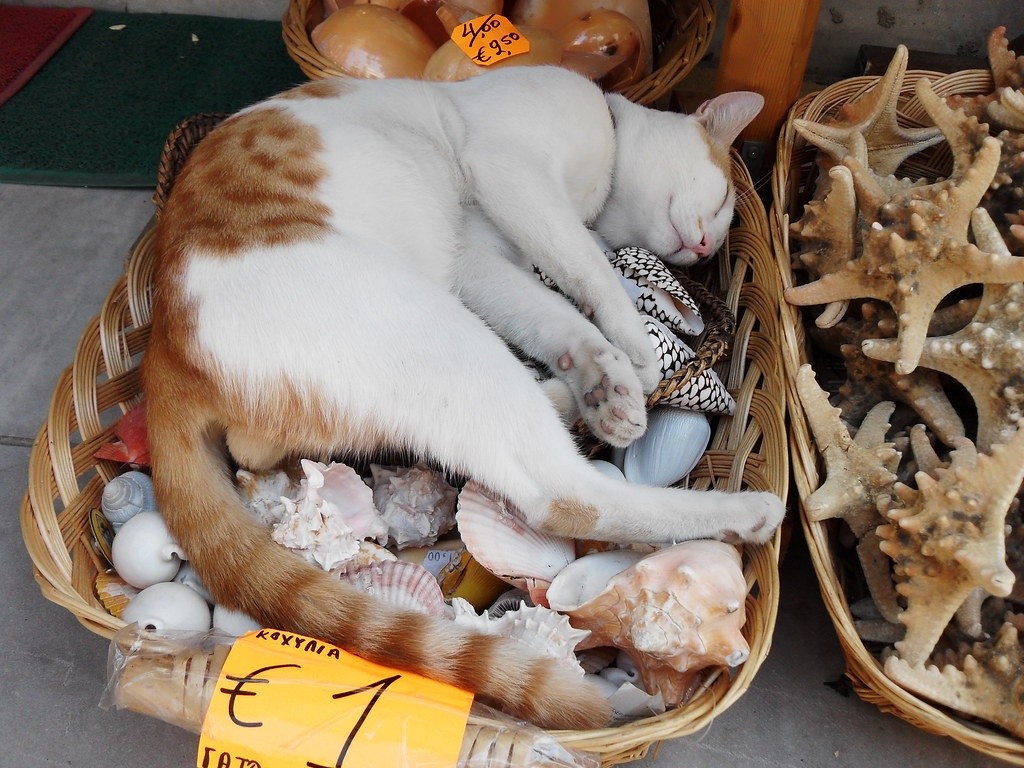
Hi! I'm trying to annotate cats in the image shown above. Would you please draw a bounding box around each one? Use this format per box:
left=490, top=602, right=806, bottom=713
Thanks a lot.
left=141, top=66, right=787, bottom=736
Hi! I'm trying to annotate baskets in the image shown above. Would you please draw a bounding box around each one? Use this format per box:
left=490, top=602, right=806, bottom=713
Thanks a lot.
left=282, top=0, right=717, bottom=107
left=21, top=115, right=800, bottom=764
left=771, top=69, right=1024, bottom=766
